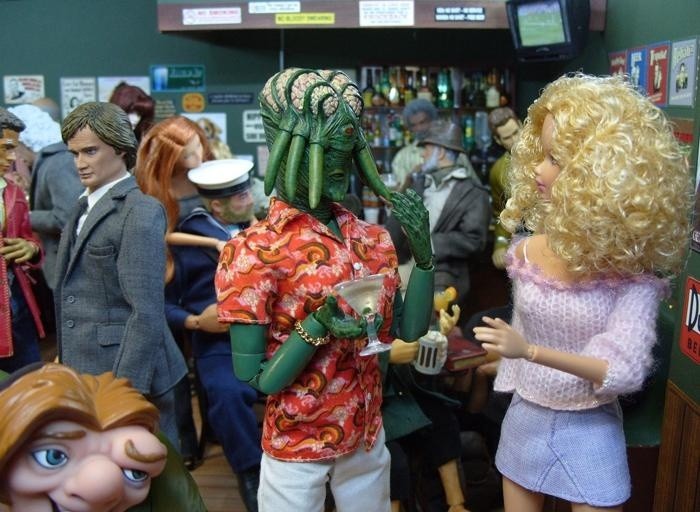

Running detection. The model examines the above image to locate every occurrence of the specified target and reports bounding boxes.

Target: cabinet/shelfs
[359,104,496,158]
